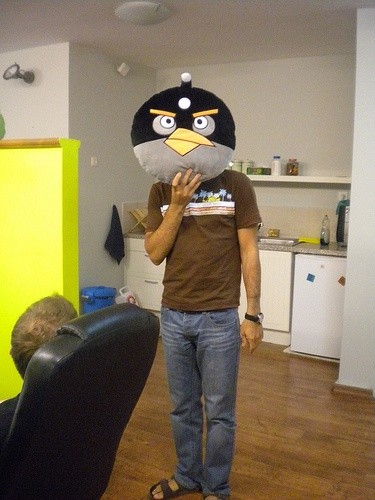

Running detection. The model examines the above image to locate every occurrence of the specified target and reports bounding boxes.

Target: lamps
[114,0,169,24]
[3,64,34,83]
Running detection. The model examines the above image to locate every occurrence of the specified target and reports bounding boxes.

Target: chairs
[0,303,161,500]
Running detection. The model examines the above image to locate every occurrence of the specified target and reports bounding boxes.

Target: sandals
[149,475,193,500]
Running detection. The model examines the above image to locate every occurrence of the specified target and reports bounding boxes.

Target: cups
[287,162,298,176]
[231,160,242,172]
[247,168,271,175]
[242,161,252,175]
[269,229,279,237]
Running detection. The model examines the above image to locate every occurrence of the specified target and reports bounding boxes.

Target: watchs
[245,312,264,325]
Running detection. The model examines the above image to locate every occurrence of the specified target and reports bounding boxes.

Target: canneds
[286,158,298,175]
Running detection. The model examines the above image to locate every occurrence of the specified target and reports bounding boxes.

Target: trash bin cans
[81,286,117,313]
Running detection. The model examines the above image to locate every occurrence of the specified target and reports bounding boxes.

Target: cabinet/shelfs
[123,237,165,310]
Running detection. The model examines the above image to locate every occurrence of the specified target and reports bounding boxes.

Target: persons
[144,169,264,500]
[0,293,78,458]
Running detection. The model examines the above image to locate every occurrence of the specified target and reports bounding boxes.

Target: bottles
[271,155,282,176]
[320,215,330,245]
